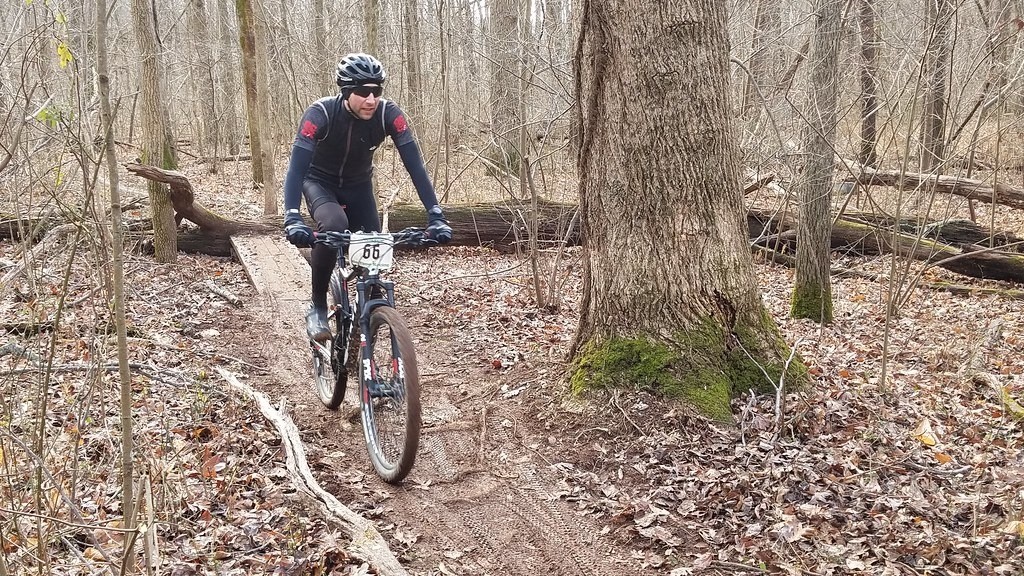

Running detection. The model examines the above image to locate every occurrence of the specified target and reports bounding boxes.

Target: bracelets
[285,208,300,216]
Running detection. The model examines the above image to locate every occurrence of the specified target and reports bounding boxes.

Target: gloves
[285,220,314,248]
[425,220,453,243]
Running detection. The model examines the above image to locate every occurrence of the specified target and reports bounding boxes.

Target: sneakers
[305,308,333,340]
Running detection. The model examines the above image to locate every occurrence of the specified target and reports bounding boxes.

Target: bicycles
[305,227,445,482]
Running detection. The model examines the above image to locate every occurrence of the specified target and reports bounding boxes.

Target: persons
[282,51,452,340]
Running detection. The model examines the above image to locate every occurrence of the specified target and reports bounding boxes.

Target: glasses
[340,85,383,97]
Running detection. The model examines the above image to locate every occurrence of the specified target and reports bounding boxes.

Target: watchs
[429,205,445,215]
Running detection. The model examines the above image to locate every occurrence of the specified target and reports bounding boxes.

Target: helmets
[336,52,386,85]
